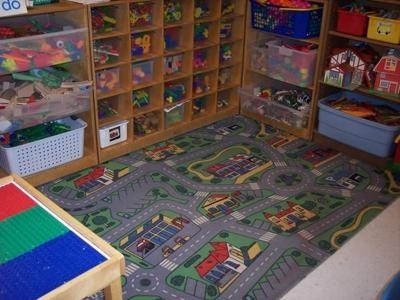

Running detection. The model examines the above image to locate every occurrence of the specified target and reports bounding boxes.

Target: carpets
[34,112,400,300]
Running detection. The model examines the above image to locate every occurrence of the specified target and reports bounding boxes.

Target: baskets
[250,0,322,40]
[0,114,88,178]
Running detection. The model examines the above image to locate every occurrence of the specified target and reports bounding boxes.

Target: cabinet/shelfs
[0,0,97,192]
[239,0,400,166]
[86,0,246,164]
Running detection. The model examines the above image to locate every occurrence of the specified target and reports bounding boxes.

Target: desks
[1,171,125,300]
